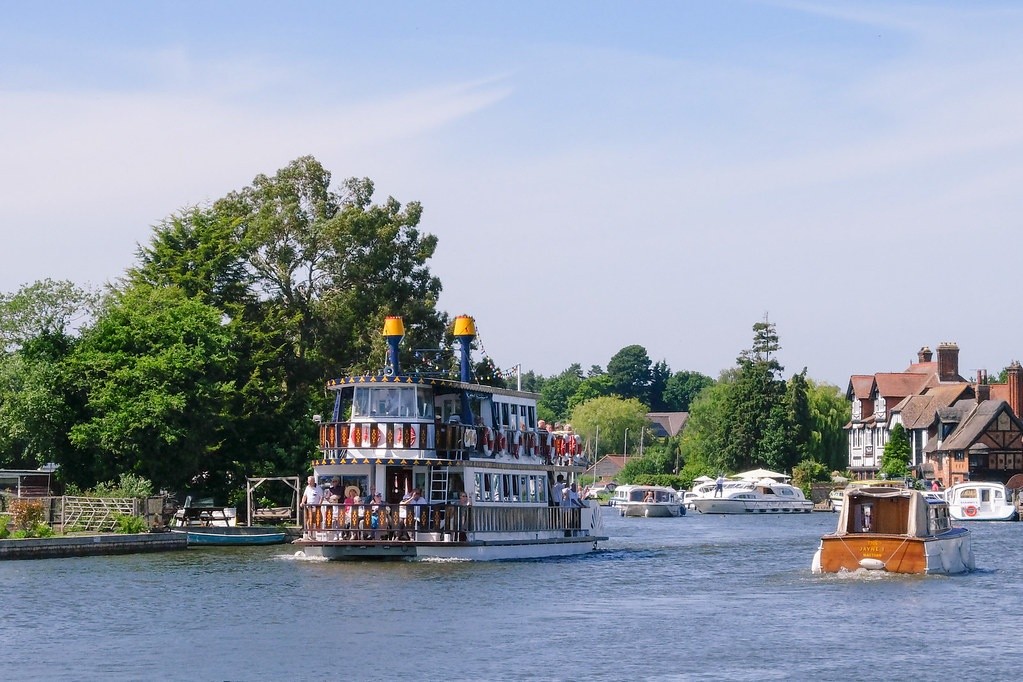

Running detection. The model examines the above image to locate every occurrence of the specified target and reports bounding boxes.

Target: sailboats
[583,424,610,499]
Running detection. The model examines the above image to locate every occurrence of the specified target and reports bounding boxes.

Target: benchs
[174,516,235,521]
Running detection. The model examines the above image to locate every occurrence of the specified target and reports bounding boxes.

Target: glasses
[461,496,467,497]
[378,496,381,497]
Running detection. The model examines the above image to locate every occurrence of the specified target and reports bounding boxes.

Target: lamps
[1004,465,1008,471]
[879,473,888,481]
[313,414,321,423]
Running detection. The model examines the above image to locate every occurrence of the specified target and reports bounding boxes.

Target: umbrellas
[729,468,790,482]
[693,476,713,492]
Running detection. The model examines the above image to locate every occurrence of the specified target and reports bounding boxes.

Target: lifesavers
[560,433,571,462]
[965,505,977,517]
[513,430,524,460]
[573,434,582,461]
[483,426,495,457]
[497,427,507,458]
[529,432,539,460]
[545,431,558,464]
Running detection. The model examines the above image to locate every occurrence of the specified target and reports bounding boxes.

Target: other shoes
[350,534,356,540]
[333,537,338,541]
[339,537,343,541]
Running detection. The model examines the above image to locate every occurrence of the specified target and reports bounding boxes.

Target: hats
[330,495,340,499]
[374,492,381,496]
[345,486,360,498]
[331,476,340,481]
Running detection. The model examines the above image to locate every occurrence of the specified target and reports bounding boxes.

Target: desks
[181,507,230,527]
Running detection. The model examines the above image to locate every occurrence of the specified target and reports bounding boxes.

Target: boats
[829,482,948,513]
[609,485,685,518]
[185,531,285,545]
[812,486,974,574]
[691,478,815,514]
[678,476,718,510]
[946,481,1015,521]
[293,313,610,560]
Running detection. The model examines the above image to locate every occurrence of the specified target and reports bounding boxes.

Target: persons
[552,473,586,527]
[535,420,573,464]
[932,481,939,491]
[714,475,723,498]
[644,492,655,503]
[298,473,470,540]
